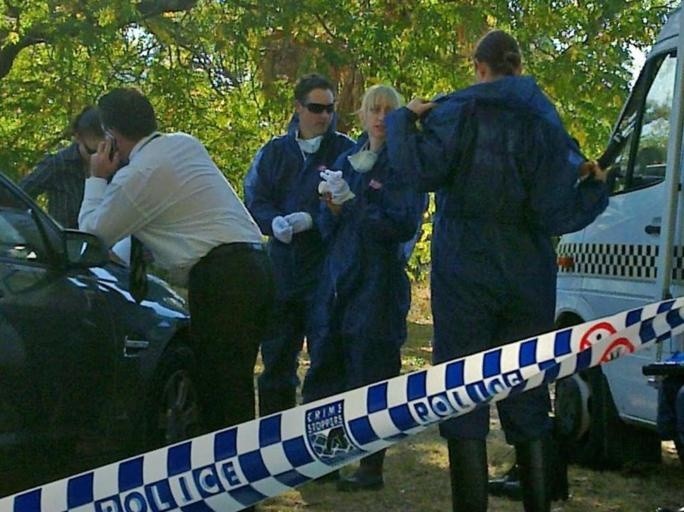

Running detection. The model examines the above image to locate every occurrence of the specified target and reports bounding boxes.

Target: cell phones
[104,131,116,157]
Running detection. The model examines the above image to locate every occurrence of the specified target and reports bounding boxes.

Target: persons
[299,84,432,492]
[384,30,609,511]
[76,89,271,433]
[18,105,107,229]
[245,74,357,482]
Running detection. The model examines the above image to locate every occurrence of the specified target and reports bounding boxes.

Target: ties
[129,235,149,307]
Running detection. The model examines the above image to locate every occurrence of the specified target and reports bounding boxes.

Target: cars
[1,170,195,460]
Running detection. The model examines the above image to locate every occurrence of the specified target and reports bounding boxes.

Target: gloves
[318,170,356,204]
[272,212,313,245]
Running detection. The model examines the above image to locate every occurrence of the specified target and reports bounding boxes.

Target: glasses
[81,141,97,155]
[301,103,334,114]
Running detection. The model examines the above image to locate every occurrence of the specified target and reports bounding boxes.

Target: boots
[315,448,385,492]
[448,438,487,511]
[518,440,550,511]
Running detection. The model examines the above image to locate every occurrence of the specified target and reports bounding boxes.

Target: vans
[551,0,683,468]
